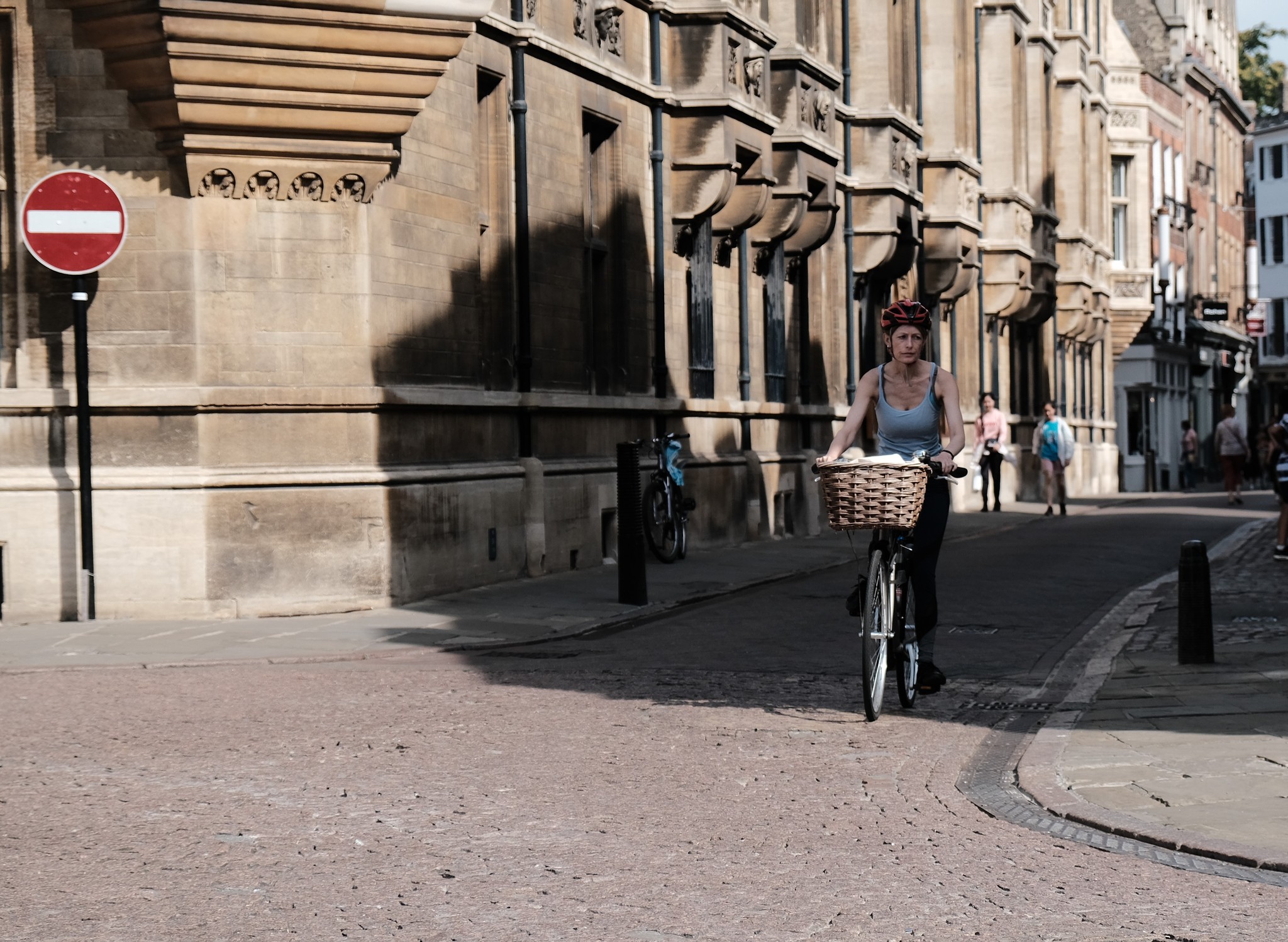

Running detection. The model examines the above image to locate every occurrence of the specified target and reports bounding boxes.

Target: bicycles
[632,434,691,563]
[812,459,969,720]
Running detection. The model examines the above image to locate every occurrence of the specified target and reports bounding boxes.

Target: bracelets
[942,450,954,459]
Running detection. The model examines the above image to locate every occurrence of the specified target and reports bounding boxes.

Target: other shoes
[916,658,946,693]
[845,575,867,617]
[1060,505,1066,516]
[992,502,1001,512]
[1045,506,1053,516]
[1273,546,1288,559]
[980,505,988,512]
[1235,498,1243,504]
[1228,499,1234,504]
[1181,484,1195,489]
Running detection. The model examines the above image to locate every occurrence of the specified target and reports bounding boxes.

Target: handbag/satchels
[1031,455,1040,471]
[985,439,998,450]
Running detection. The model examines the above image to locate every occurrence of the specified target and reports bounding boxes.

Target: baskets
[817,460,933,531]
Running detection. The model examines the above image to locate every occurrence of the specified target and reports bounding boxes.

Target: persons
[817,299,965,686]
[1179,404,1288,560]
[1031,401,1074,517]
[973,392,1007,512]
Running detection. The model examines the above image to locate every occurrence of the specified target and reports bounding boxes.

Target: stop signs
[19,169,129,275]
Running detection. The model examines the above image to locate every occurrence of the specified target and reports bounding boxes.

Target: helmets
[881,299,932,333]
